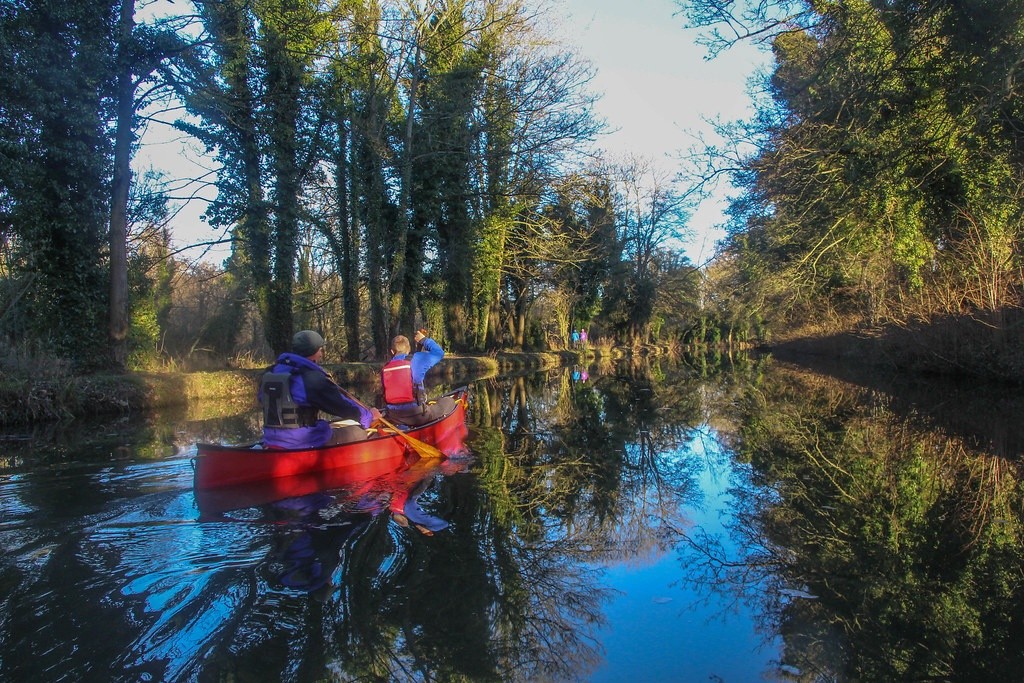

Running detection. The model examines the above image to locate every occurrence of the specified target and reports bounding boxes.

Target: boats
[192,385,470,514]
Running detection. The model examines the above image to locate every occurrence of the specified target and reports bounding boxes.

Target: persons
[257,330,382,450]
[381,331,454,426]
[580,328,587,350]
[572,329,580,349]
[580,372,589,384]
[572,371,581,383]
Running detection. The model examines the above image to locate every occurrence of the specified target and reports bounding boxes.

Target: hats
[291,330,328,356]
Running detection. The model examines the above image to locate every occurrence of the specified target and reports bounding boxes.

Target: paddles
[335,382,449,459]
[416,329,428,352]
